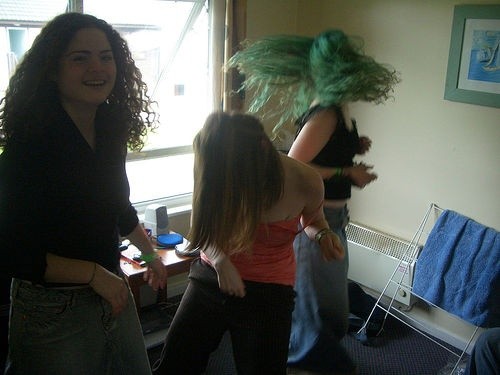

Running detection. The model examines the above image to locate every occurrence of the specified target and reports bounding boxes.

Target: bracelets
[87,263,96,284]
[315,228,333,245]
[335,168,343,177]
[139,252,157,265]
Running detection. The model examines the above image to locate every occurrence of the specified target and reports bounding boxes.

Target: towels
[411,207,500,330]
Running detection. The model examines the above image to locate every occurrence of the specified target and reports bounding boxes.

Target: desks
[116,215,197,318]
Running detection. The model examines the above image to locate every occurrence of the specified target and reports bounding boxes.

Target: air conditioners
[343,221,424,306]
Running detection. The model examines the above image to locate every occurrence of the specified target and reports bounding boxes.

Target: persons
[220,27,402,367]
[0,12,168,374]
[153,110,345,375]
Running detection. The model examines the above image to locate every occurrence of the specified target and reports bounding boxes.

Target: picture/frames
[443,4,500,110]
